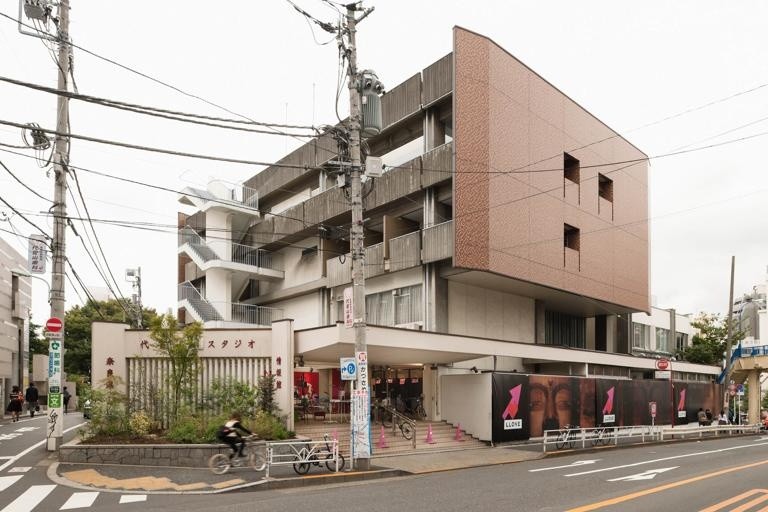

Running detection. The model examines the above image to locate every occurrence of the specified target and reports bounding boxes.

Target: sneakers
[229,454,248,460]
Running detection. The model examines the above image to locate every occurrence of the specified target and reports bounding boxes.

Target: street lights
[736,298,767,357]
[9,3,70,452]
[126,264,144,328]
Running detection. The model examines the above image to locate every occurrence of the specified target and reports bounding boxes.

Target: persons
[217,409,258,460]
[697,407,714,426]
[528,383,574,436]
[718,410,728,434]
[26,382,39,417]
[6,385,24,422]
[63,386,72,413]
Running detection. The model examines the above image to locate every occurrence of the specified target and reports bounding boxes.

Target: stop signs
[46,318,62,332]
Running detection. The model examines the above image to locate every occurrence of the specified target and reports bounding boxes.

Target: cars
[759,406,768,430]
[83,399,92,418]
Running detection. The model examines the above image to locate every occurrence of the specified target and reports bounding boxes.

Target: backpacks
[216,426,230,437]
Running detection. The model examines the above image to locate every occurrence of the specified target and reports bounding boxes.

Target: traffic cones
[426,424,436,444]
[375,425,390,448]
[453,422,464,441]
[328,428,341,452]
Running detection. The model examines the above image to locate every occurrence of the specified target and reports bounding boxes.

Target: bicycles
[209,434,265,473]
[590,422,612,446]
[399,392,426,420]
[556,424,577,449]
[293,433,345,474]
[383,407,415,441]
